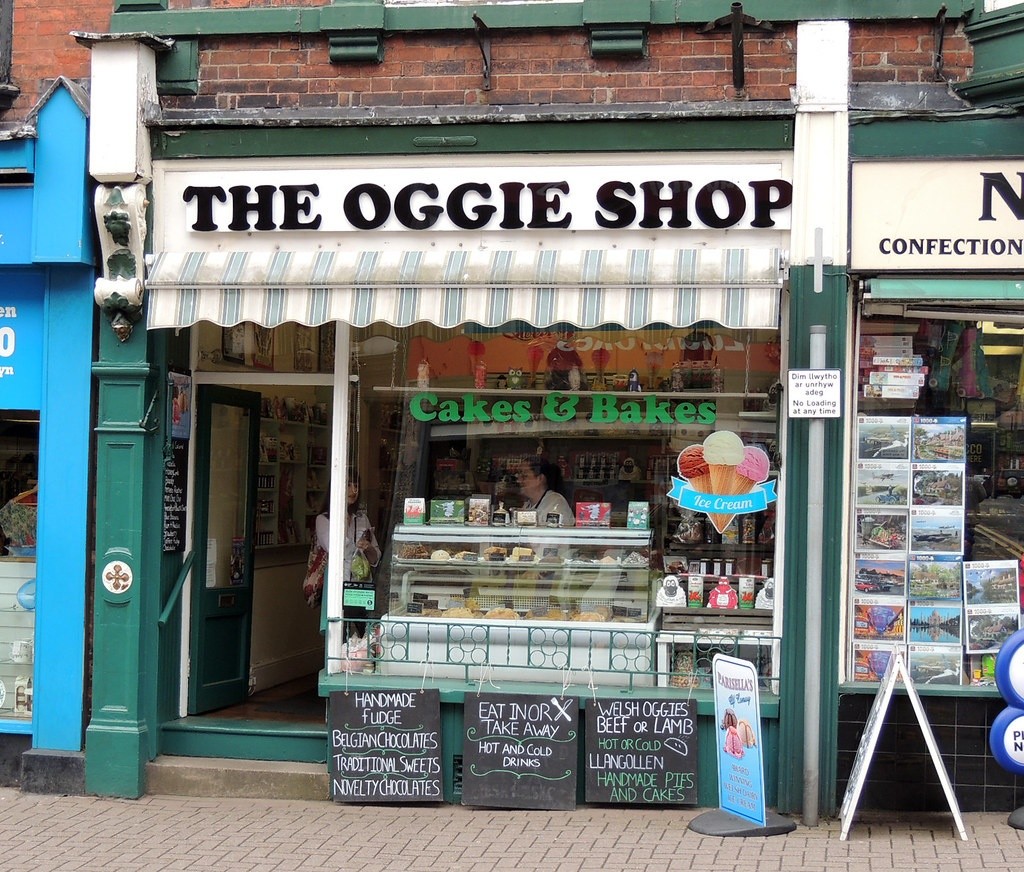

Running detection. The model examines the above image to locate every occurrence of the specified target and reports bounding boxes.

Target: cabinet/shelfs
[236,372,1024,695]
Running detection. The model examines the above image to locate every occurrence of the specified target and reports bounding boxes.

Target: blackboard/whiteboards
[584,697,700,806]
[329,689,444,802]
[461,692,579,810]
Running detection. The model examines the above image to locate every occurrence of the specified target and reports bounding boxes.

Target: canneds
[700,558,773,578]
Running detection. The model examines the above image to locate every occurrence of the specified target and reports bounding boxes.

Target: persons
[516,454,574,560]
[315,466,381,642]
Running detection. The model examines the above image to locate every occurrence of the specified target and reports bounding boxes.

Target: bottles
[475,360,487,388]
[417,359,429,388]
[629,368,638,392]
[558,453,619,480]
[671,361,724,393]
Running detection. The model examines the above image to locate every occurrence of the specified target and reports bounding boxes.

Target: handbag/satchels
[302,511,329,609]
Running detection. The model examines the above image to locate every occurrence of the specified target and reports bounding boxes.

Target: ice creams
[677,431,769,534]
[721,708,756,757]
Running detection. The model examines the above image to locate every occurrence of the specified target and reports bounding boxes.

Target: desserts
[399,545,649,566]
